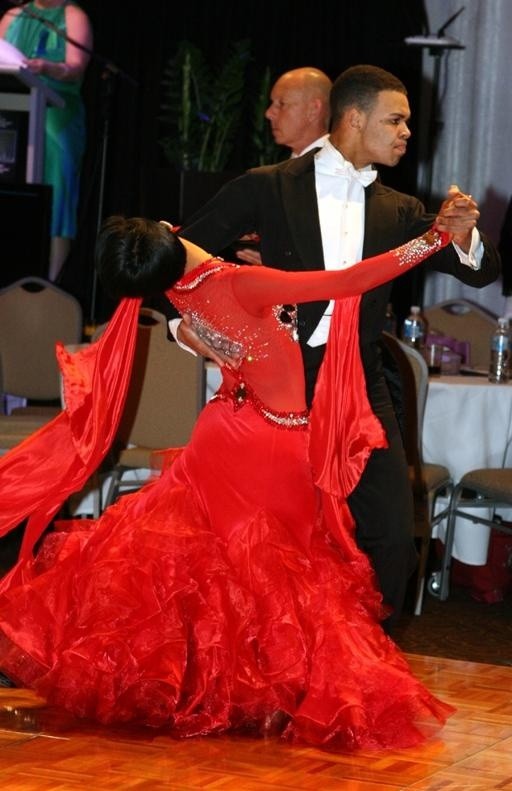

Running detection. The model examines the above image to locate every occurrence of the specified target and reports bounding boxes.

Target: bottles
[402,305,426,352]
[420,329,444,374]
[489,317,510,385]
[383,303,397,337]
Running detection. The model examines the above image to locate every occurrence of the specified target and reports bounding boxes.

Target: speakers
[0,183,52,292]
[180,171,247,225]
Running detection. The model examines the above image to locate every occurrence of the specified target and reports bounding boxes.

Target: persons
[0,0,95,284]
[0,184,479,756]
[163,65,500,630]
[235,67,333,266]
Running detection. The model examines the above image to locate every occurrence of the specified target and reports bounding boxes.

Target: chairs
[383,297,512,618]
[1,277,205,521]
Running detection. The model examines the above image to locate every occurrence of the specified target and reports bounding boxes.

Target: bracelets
[55,62,70,81]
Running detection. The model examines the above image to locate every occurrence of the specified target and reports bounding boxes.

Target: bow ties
[315,159,378,189]
